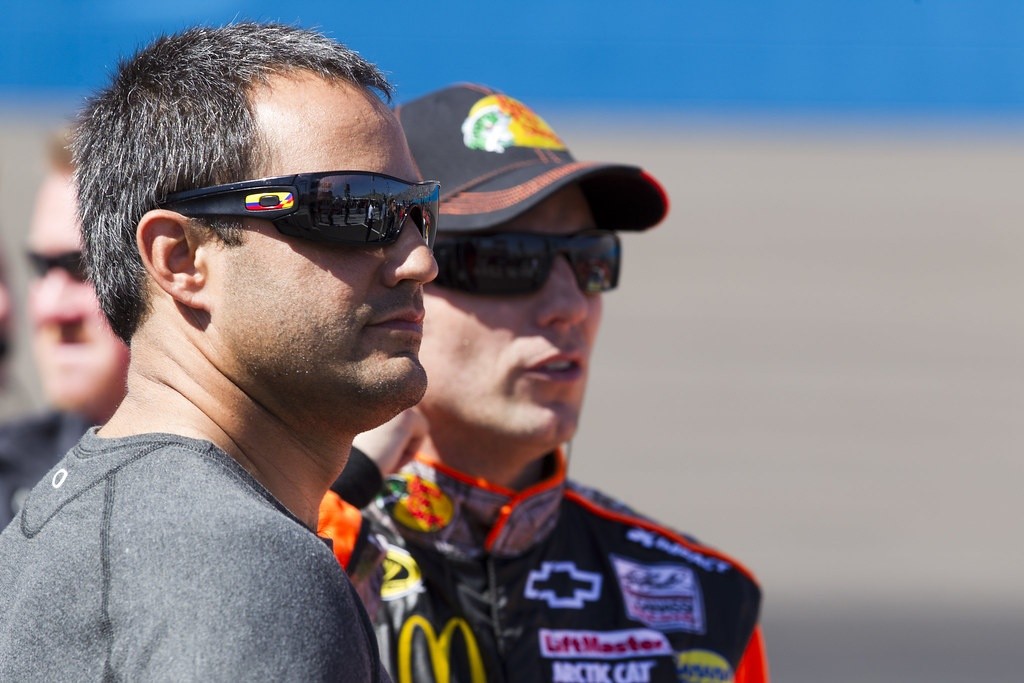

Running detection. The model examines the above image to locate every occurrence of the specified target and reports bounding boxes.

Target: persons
[0,21,439,683]
[309,200,324,227]
[344,203,350,225]
[327,200,334,226]
[364,199,410,241]
[314,84,769,683]
[575,254,610,291]
[1,121,128,530]
[531,255,539,285]
[421,202,430,239]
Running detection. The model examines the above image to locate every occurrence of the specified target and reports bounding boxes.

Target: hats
[392,85,668,235]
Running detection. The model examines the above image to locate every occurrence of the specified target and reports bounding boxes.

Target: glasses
[147,172,440,252]
[431,228,620,299]
[22,242,90,282]
